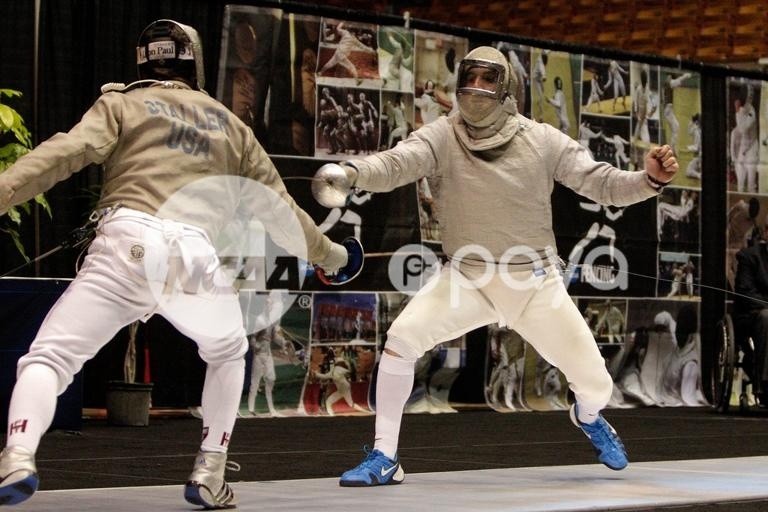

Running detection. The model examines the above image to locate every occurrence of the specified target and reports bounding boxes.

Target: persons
[735,210,768,404]
[0,17,678,509]
[653,72,759,405]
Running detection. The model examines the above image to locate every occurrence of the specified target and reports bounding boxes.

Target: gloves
[338,164,357,189]
[317,239,350,277]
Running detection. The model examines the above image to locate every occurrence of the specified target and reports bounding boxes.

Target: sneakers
[0,446,38,504]
[568,403,629,471]
[339,449,405,486]
[184,448,240,508]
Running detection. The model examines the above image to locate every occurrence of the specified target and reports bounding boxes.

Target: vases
[103,378,157,428]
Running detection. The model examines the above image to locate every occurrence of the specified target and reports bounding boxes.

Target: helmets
[135,19,206,91]
[692,113,701,122]
[541,51,549,65]
[554,76,563,90]
[455,45,517,124]
[641,71,648,88]
